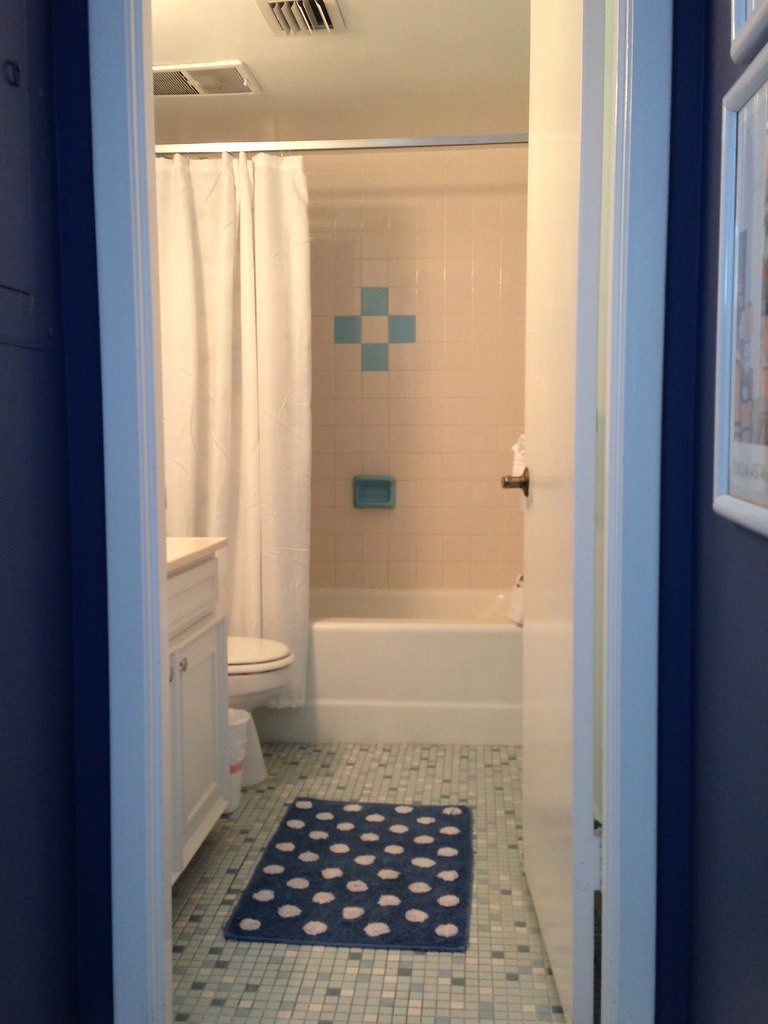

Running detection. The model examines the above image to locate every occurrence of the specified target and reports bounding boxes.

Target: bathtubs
[251,587,524,747]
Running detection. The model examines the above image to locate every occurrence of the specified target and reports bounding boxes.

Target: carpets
[221,798,474,953]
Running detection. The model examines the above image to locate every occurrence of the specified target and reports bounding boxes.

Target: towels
[507,574,525,625]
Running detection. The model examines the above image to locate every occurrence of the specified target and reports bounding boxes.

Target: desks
[166,535,230,889]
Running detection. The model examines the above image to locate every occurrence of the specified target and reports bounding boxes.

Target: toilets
[226,636,296,789]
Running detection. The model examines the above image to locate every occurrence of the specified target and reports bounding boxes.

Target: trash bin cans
[222,708,251,815]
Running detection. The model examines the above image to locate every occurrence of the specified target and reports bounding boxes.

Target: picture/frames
[711,0,768,535]
